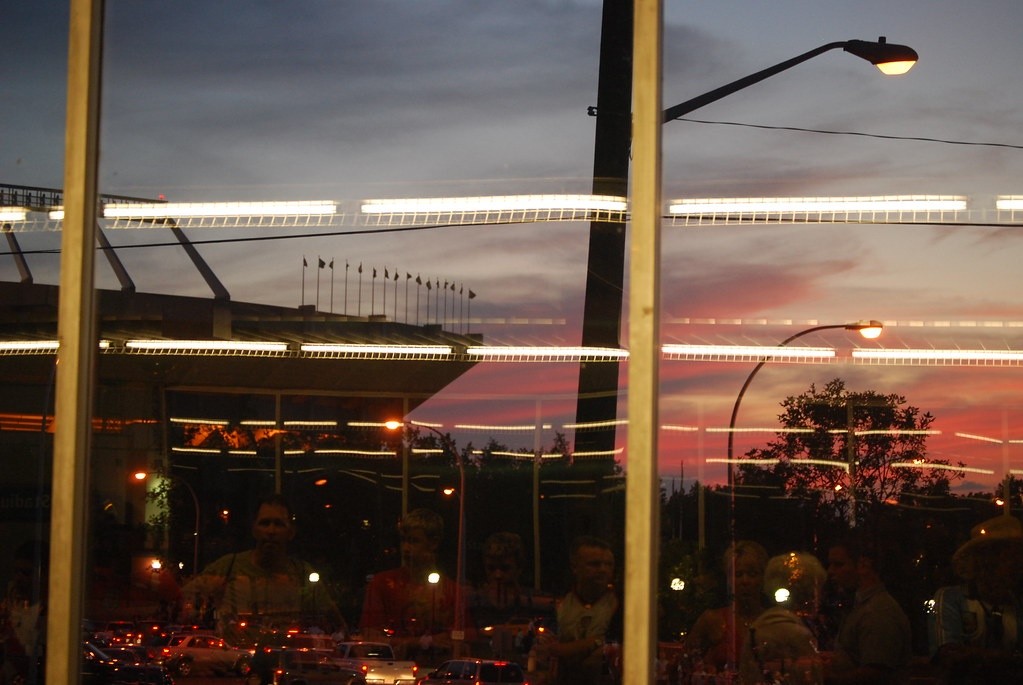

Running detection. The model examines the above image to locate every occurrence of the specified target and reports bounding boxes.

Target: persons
[0,494,1023,685]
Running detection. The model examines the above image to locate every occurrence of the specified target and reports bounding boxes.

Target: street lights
[308,572,320,625]
[571,31,921,472]
[425,570,442,663]
[384,416,465,659]
[134,466,201,608]
[724,318,885,668]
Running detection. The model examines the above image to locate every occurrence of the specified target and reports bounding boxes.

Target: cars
[82,602,688,684]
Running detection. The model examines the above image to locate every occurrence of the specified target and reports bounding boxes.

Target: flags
[304,258,399,280]
[406,274,476,298]
